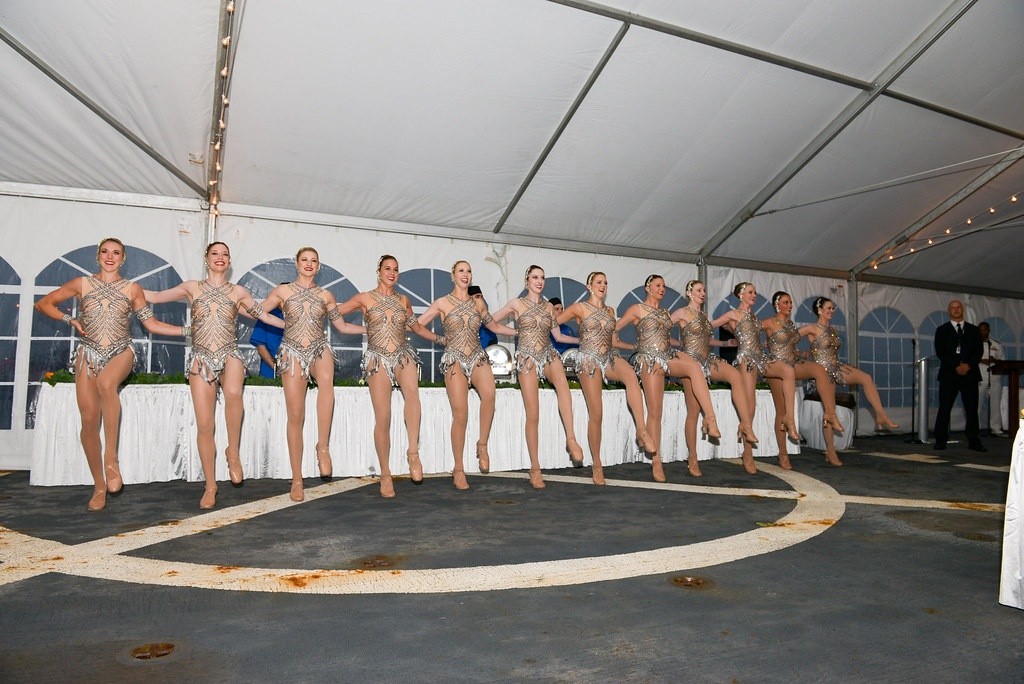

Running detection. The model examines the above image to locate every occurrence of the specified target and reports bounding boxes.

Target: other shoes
[933,430,1009,452]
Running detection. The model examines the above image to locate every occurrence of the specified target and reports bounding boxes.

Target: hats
[468,284,481,295]
[549,297,562,306]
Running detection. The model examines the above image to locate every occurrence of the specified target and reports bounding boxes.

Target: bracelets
[61,314,74,326]
[181,325,192,338]
[434,334,444,345]
[722,341,728,347]
[633,343,638,351]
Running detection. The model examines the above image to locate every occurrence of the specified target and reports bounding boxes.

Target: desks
[27,382,803,483]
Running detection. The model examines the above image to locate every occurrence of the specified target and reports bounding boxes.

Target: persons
[249,282,288,379]
[934,301,988,453]
[615,275,720,483]
[33,237,192,510]
[405,259,517,491]
[974,320,1005,438]
[711,283,803,475]
[797,297,898,466]
[491,265,581,489]
[762,288,844,470]
[673,280,759,477]
[557,270,656,486]
[466,285,499,350]
[141,242,285,510]
[549,298,580,354]
[238,248,369,501]
[338,255,446,497]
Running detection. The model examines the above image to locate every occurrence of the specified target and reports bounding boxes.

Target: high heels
[379,475,395,498]
[452,470,469,490]
[88,486,106,511]
[316,443,333,478]
[289,479,304,501]
[702,417,721,438]
[103,462,122,493]
[739,414,896,475]
[592,467,606,486]
[566,439,583,463]
[200,483,218,509]
[477,441,489,472]
[653,462,666,482]
[225,447,242,484]
[637,430,656,453]
[407,451,423,482]
[529,469,544,489]
[687,457,702,476]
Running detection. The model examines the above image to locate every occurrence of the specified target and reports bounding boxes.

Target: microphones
[986,339,991,345]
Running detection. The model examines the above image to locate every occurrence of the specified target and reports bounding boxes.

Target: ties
[957,323,963,347]
